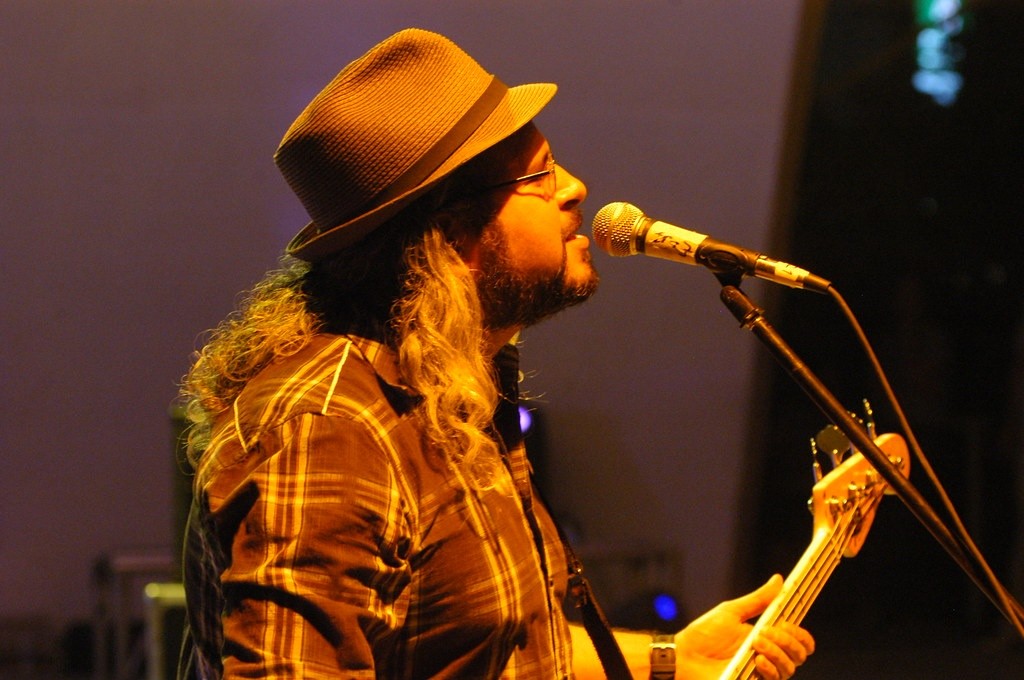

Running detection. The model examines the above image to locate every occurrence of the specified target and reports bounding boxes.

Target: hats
[272,26,559,265]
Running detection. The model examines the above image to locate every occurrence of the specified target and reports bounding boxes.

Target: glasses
[433,160,557,217]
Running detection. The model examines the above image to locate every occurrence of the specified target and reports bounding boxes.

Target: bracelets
[648,632,676,680]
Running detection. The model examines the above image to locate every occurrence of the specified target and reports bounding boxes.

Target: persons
[174,28,815,680]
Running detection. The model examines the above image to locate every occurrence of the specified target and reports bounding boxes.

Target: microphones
[591,200,834,294]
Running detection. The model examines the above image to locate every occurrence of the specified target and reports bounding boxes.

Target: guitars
[718,397,913,680]
